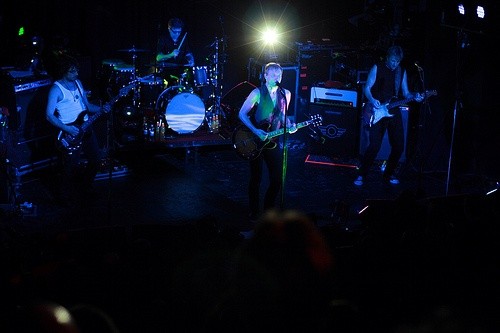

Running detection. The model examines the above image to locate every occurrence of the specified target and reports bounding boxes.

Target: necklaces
[62,79,76,102]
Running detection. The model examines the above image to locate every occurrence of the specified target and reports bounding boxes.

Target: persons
[154,17,195,67]
[355,44,423,187]
[238,63,292,209]
[0,210,500,333]
[46,53,112,208]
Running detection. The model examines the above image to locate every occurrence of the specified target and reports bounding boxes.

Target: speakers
[2,86,56,164]
[305,103,359,161]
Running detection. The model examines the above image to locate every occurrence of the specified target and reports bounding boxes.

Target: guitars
[232,114,324,161]
[56,80,138,157]
[363,88,440,127]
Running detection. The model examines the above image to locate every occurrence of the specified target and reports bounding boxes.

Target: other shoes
[250,211,256,223]
[352,175,366,188]
[384,175,400,186]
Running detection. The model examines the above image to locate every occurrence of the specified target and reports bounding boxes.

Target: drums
[184,66,209,89]
[155,85,206,135]
[136,79,162,103]
[103,60,125,75]
[115,67,136,86]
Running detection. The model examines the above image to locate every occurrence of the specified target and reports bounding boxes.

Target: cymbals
[143,63,183,68]
[115,48,153,52]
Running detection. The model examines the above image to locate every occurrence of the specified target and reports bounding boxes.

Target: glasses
[67,68,80,74]
[170,28,182,34]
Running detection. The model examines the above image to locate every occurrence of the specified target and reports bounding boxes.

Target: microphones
[276,81,285,93]
[414,61,425,72]
